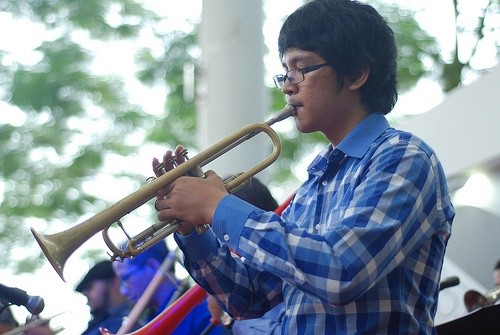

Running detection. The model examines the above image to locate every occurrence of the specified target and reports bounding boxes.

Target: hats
[74,259,115,292]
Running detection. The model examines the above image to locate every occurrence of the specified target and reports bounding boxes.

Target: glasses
[274,62,327,90]
[120,265,139,280]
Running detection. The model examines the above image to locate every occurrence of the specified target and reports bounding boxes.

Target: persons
[491,260,500,285]
[0,172,283,335]
[152,0,455,335]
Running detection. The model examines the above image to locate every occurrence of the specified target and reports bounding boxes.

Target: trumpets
[25,103,297,282]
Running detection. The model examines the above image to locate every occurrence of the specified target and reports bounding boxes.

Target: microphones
[440,276,460,291]
[0,283,45,316]
